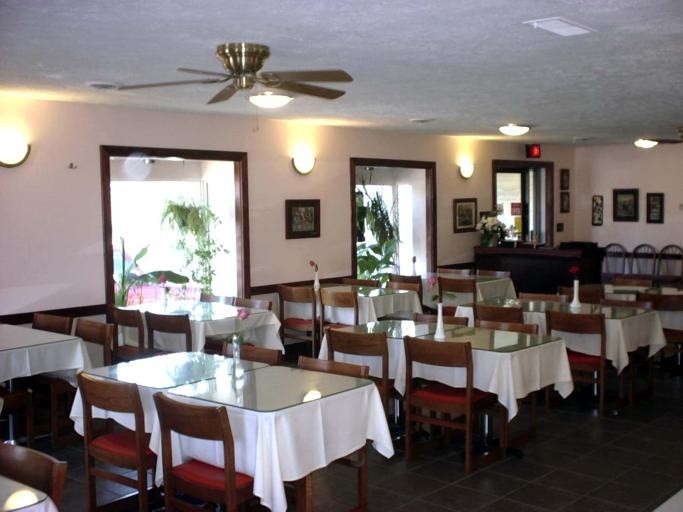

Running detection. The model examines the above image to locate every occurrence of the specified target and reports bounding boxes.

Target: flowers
[475,214,501,246]
[309,260,317,268]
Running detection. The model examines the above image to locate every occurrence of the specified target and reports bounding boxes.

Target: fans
[118,41,354,105]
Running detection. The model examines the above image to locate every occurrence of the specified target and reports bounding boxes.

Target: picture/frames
[646,192,664,223]
[612,188,639,222]
[453,197,477,233]
[561,169,569,190]
[592,194,603,226]
[560,192,569,213]
[285,198,320,238]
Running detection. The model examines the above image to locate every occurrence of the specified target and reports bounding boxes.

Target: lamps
[499,123,531,136]
[634,138,659,149]
[292,144,316,175]
[0,127,31,167]
[459,159,475,179]
[246,89,294,109]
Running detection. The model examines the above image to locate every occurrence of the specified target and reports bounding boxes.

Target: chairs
[629,244,656,275]
[600,242,626,282]
[657,245,683,276]
